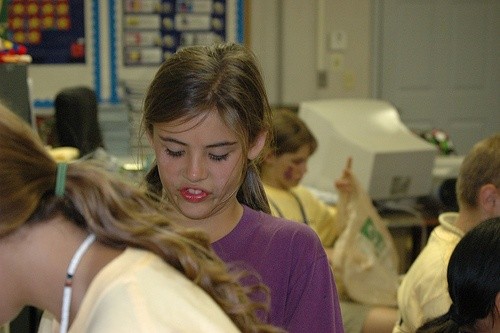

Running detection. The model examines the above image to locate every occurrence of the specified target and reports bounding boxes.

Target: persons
[0,43,500,333]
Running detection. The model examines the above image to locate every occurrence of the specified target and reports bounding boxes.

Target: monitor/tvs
[295,100,436,199]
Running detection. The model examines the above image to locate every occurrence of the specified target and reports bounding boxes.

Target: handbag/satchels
[333,171,399,308]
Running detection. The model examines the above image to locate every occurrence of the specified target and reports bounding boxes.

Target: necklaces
[264,189,308,225]
[59,234,95,333]
